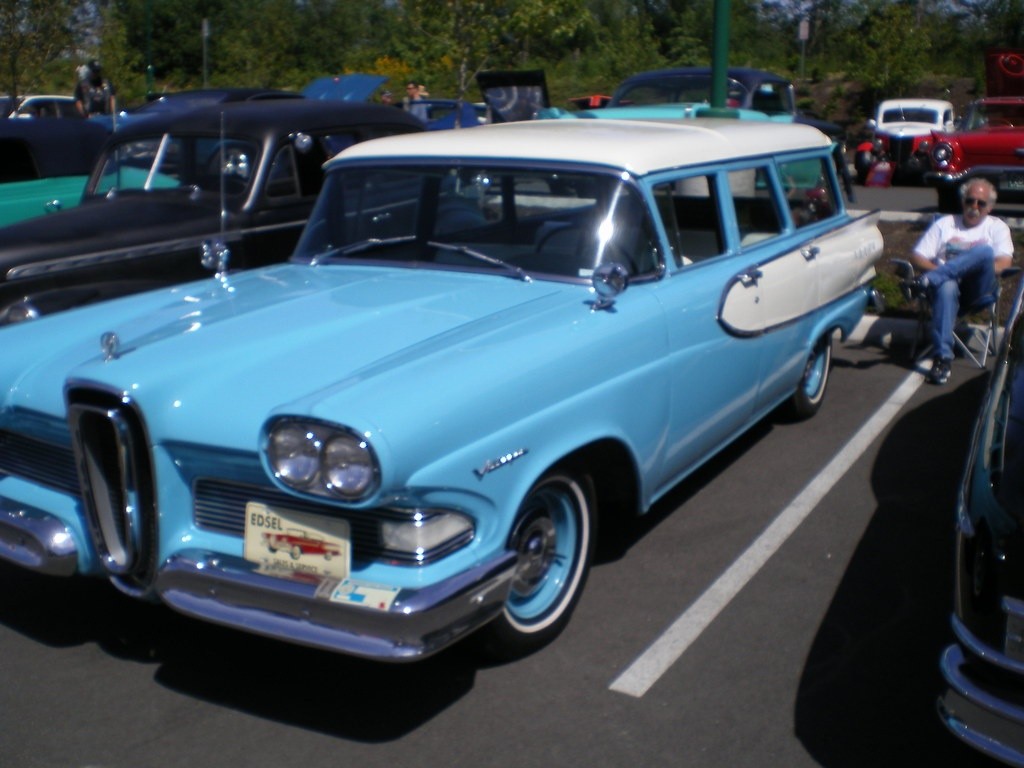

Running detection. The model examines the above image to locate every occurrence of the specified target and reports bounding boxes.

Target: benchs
[535,220,776,264]
[435,243,644,279]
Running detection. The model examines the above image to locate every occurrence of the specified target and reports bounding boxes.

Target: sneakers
[898,277,923,304]
[926,357,951,384]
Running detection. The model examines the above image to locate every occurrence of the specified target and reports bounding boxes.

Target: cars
[932,274,1024,768]
[913,95,1024,209]
[851,93,960,189]
[0,59,852,331]
[0,109,888,668]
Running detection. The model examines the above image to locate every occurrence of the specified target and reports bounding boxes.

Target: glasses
[965,198,986,209]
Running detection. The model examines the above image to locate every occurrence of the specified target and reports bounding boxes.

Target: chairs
[887,212,1024,371]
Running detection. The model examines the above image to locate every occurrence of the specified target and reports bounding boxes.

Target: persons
[380,82,430,122]
[76,61,117,120]
[896,180,1014,385]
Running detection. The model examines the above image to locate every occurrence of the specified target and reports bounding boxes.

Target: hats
[88,60,101,71]
[380,90,391,97]
[418,85,429,96]
